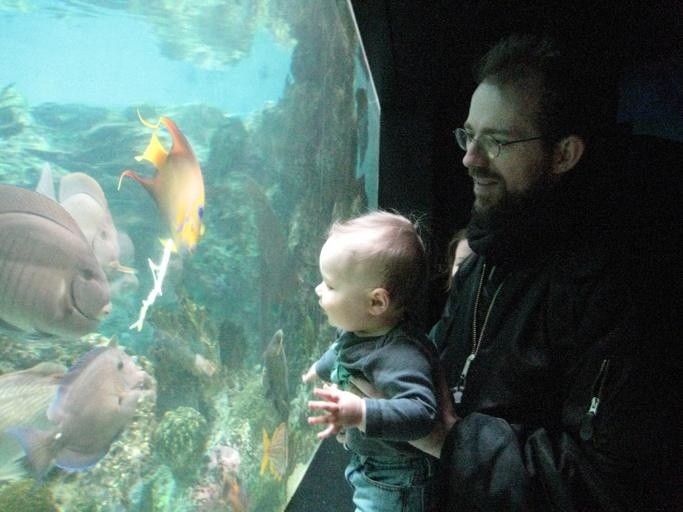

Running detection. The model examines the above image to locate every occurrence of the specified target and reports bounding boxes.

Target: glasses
[455,127,543,159]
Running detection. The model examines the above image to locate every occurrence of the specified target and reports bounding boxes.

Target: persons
[447,224,474,280]
[301,209,443,511]
[346,30,681,512]
